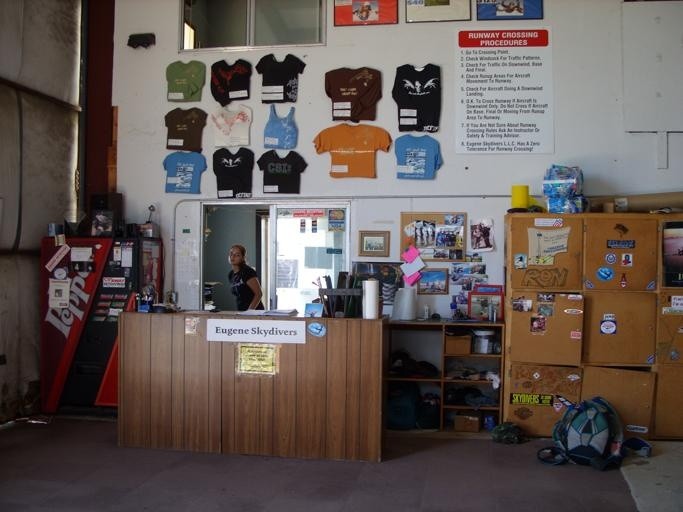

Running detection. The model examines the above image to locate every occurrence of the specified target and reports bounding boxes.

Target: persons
[435,230,462,246]
[227,244,265,311]
[473,224,487,247]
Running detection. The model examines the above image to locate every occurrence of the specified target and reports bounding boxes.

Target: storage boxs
[445,330,471,353]
[470,326,494,355]
[455,410,483,431]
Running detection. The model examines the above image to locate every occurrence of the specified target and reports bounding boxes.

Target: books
[239,306,299,316]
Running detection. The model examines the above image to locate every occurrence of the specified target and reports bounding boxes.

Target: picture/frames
[468,292,503,319]
[474,284,503,293]
[417,268,448,295]
[357,229,389,258]
[332,0,544,28]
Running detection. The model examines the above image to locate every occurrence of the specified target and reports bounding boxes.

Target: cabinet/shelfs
[501,209,681,443]
[384,318,504,440]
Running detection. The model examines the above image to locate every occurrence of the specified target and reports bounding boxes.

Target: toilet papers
[393,288,418,321]
[361,280,380,320]
